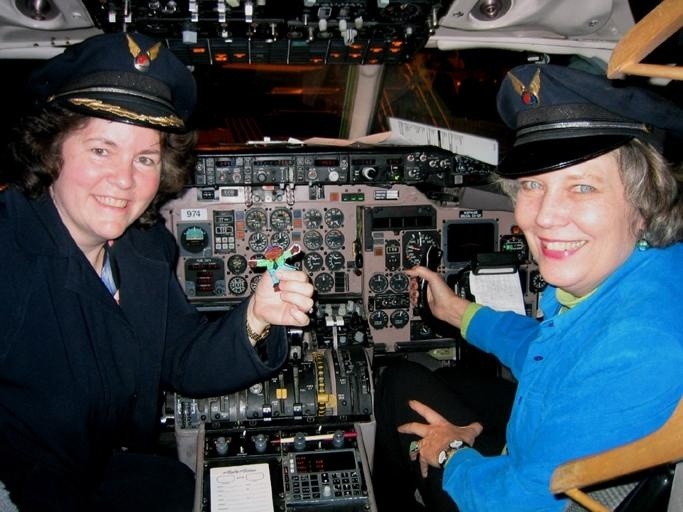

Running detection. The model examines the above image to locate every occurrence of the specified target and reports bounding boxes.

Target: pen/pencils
[270,431,357,443]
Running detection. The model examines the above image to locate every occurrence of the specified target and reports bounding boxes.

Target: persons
[0,31,314,512]
[394,60,683,512]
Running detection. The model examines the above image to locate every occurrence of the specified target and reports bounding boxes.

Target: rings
[409,440,419,453]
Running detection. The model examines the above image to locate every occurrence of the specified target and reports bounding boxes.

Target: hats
[26,31,199,135]
[493,62,682,179]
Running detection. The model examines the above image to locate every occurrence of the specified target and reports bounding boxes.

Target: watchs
[437,448,451,469]
[445,438,471,451]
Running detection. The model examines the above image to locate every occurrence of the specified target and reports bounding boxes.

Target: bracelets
[245,321,271,341]
